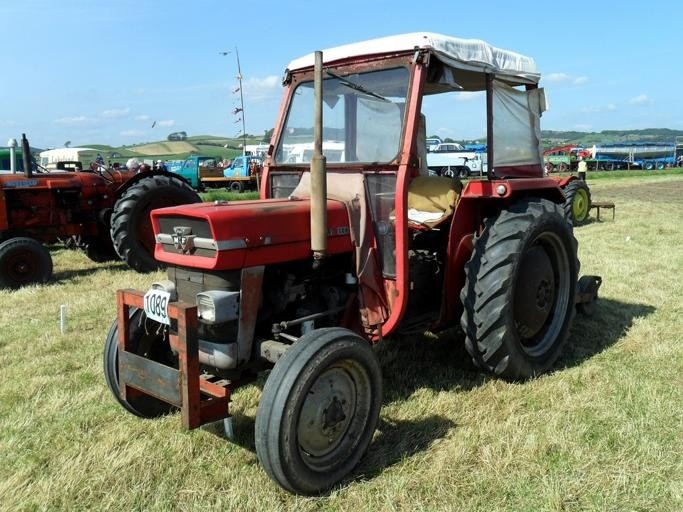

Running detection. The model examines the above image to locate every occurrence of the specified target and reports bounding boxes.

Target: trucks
[571,143,678,171]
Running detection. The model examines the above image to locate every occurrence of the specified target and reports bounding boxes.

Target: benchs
[589,202,615,220]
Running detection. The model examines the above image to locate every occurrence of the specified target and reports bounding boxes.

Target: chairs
[388,176,463,231]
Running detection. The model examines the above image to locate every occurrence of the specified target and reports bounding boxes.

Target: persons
[89,154,257,180]
[544,163,549,176]
[577,156,587,180]
